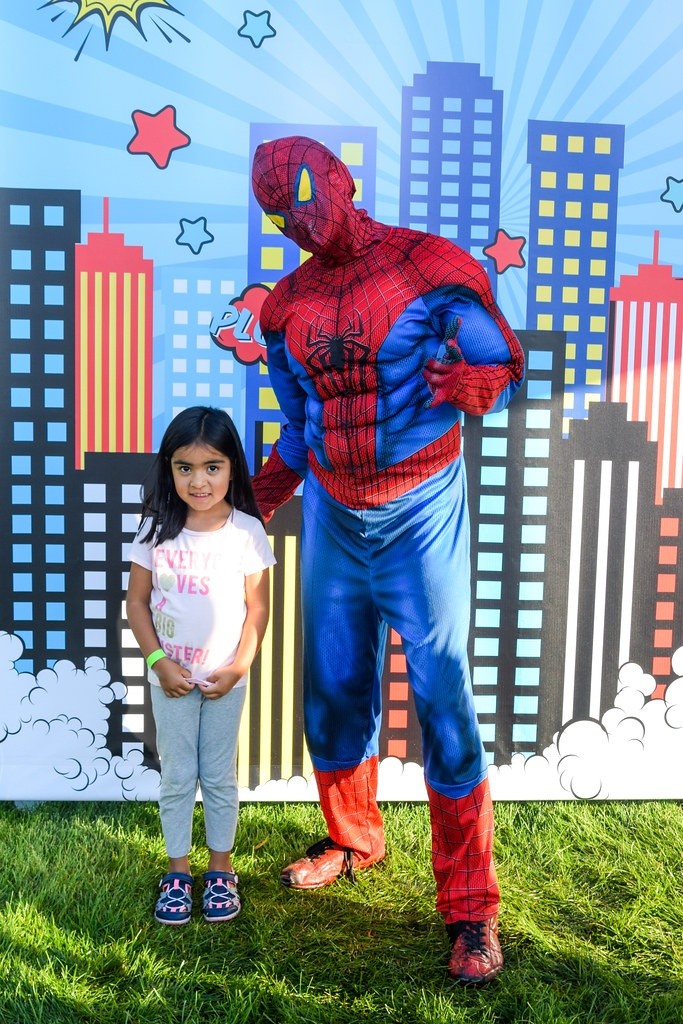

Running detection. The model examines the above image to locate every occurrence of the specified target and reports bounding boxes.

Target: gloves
[420,316,467,410]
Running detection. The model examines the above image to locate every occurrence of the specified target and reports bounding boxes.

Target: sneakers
[280,836,387,889]
[447,911,504,984]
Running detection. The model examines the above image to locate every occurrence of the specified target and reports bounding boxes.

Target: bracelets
[146,649,166,669]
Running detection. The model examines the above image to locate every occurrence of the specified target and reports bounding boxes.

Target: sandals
[154,872,195,925]
[201,866,242,921]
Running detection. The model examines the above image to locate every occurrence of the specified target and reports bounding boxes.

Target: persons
[124,406,277,925]
[252,137,525,987]
